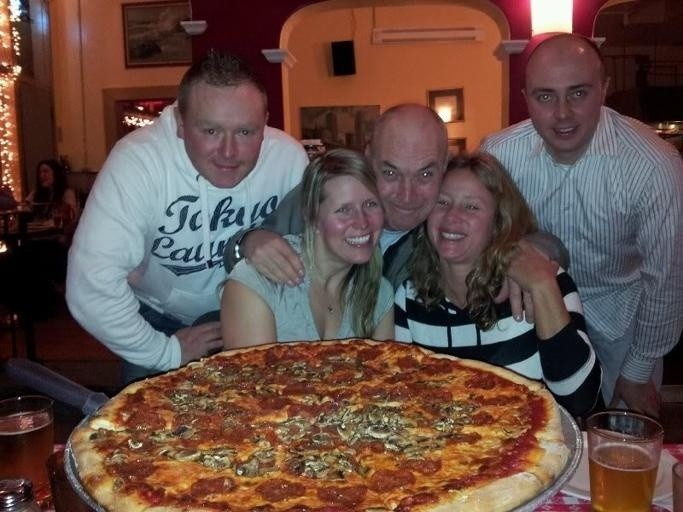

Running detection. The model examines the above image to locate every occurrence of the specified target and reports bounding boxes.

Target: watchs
[235,233,247,263]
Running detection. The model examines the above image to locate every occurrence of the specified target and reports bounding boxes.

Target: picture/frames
[427,89,465,125]
[102,85,178,158]
[447,138,467,159]
[122,1,193,69]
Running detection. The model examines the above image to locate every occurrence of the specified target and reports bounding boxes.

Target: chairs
[0,212,40,364]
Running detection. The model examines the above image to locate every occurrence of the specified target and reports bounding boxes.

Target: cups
[586,411,663,512]
[0,397,56,508]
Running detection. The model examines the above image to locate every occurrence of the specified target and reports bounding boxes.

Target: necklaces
[312,278,347,315]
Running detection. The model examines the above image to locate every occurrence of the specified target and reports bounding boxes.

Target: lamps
[372,26,481,42]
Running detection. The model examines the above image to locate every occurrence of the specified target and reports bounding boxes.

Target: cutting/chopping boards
[6,346,581,512]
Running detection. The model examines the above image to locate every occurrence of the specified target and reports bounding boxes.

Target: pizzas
[69,337,571,512]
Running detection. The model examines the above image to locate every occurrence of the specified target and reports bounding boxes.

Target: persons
[393,150,604,421]
[22,157,81,218]
[472,33,683,434]
[225,101,570,323]
[220,148,394,353]
[64,47,311,388]
